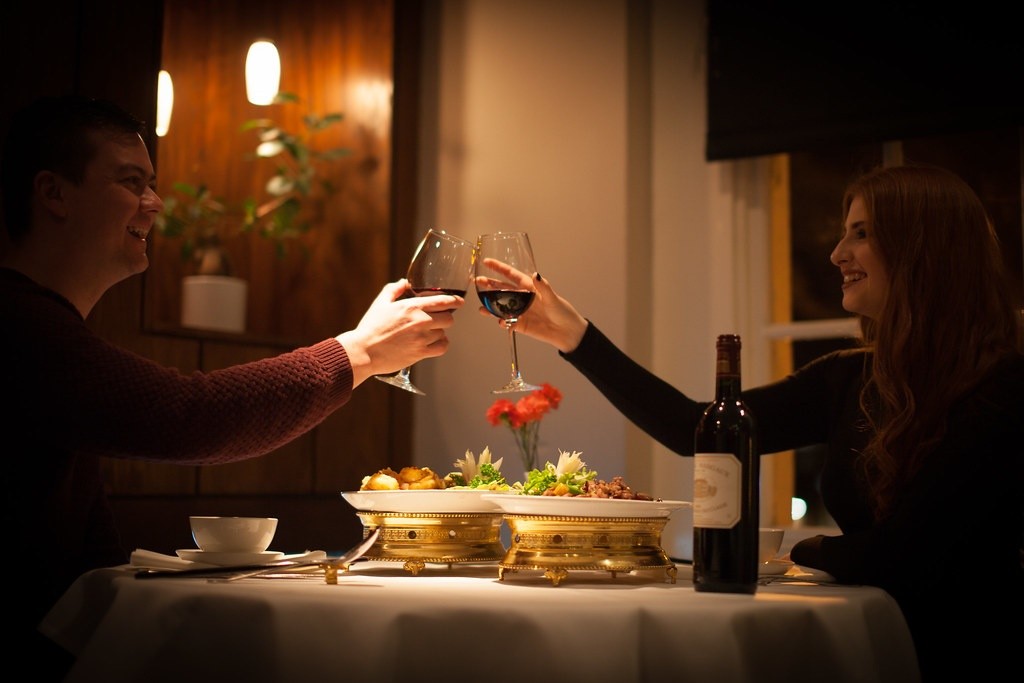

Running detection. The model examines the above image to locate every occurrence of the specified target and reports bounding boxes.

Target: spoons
[136,527,382,579]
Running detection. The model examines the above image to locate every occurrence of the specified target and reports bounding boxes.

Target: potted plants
[153,93,379,330]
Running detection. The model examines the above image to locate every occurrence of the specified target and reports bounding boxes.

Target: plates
[176,549,285,566]
[758,561,795,575]
[481,493,692,518]
[342,491,519,512]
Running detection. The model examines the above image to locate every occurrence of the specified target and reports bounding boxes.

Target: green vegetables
[468,464,506,490]
[517,460,598,496]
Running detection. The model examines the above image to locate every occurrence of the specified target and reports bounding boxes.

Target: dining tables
[62,561,917,683]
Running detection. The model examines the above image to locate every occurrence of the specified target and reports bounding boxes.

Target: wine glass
[375,229,478,396]
[475,232,544,394]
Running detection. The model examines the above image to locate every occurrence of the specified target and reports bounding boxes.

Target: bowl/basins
[190,516,278,552]
[759,528,784,562]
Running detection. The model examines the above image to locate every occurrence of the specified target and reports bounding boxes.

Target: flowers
[487,383,562,474]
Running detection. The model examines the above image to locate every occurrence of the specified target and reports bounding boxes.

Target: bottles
[693,334,761,593]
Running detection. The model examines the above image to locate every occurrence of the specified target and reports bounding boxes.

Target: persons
[479,160,1024,683]
[0,90,467,683]
